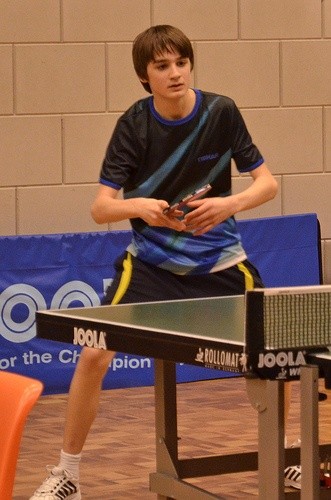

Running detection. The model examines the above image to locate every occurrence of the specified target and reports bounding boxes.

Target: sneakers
[285,439,331,490]
[29,465,82,500]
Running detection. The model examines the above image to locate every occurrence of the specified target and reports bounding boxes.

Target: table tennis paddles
[163,183,215,217]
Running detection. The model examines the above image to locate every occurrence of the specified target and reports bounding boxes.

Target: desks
[35,285,331,500]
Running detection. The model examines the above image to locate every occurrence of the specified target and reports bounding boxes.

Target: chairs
[0,370,44,500]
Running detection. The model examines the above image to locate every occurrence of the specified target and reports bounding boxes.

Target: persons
[30,24,331,500]
